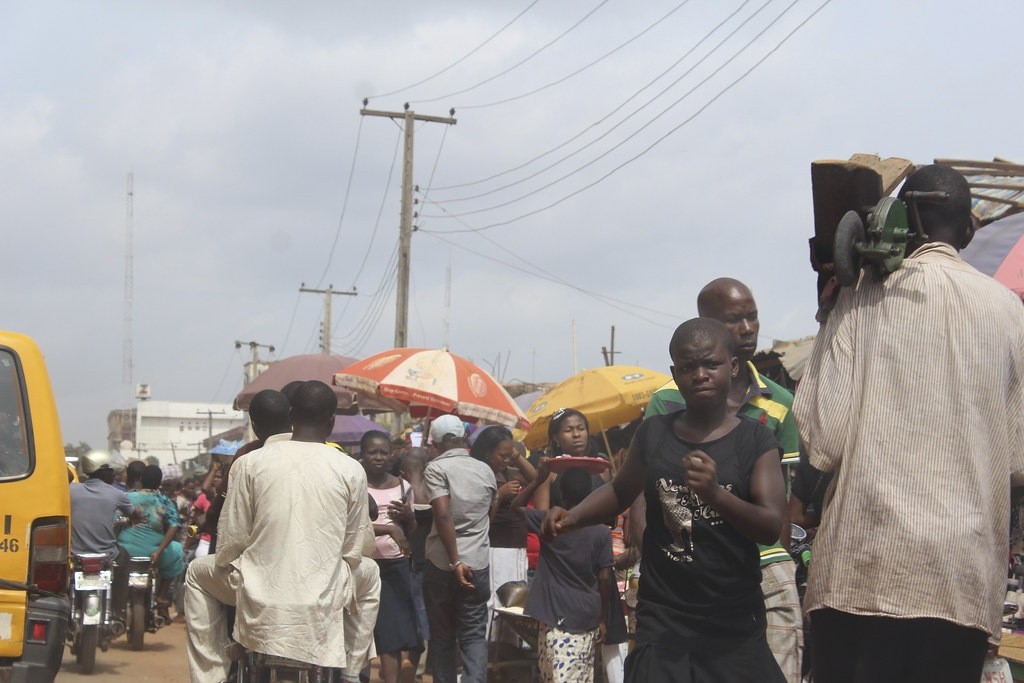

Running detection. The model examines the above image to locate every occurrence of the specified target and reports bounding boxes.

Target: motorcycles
[231,605,335,683]
[68,549,126,674]
[126,555,164,652]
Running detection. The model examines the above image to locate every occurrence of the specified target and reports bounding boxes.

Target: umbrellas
[959,212,1024,300]
[332,346,532,448]
[511,366,672,476]
[326,415,389,443]
[232,354,395,416]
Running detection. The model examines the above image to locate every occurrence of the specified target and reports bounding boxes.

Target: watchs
[449,561,462,570]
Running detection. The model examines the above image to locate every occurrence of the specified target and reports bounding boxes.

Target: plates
[546,457,610,475]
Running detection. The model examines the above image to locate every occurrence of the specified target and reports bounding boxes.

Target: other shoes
[399,660,416,683]
[152,596,171,604]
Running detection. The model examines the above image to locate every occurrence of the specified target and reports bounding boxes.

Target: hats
[430,414,465,443]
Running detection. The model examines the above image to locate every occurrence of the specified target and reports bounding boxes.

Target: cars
[0,326,72,683]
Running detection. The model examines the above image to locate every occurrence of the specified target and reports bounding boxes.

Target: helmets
[80,449,117,474]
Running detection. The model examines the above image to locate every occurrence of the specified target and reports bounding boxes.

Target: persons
[114,465,183,625]
[423,414,497,683]
[792,165,1024,683]
[184,380,381,683]
[631,277,804,683]
[510,457,616,683]
[70,450,142,623]
[126,410,647,683]
[540,317,788,683]
